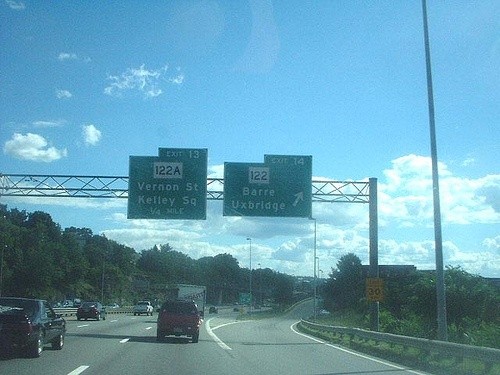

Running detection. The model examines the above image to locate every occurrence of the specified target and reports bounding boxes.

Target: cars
[157,299,200,343]
[48,297,120,311]
[77,302,106,321]
[232,299,272,312]
[209,307,218,314]
[0,297,66,358]
[134,301,153,316]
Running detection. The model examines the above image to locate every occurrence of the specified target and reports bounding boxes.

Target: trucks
[178,285,206,320]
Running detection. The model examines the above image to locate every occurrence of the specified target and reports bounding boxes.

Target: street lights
[309,218,317,323]
[246,237,251,294]
